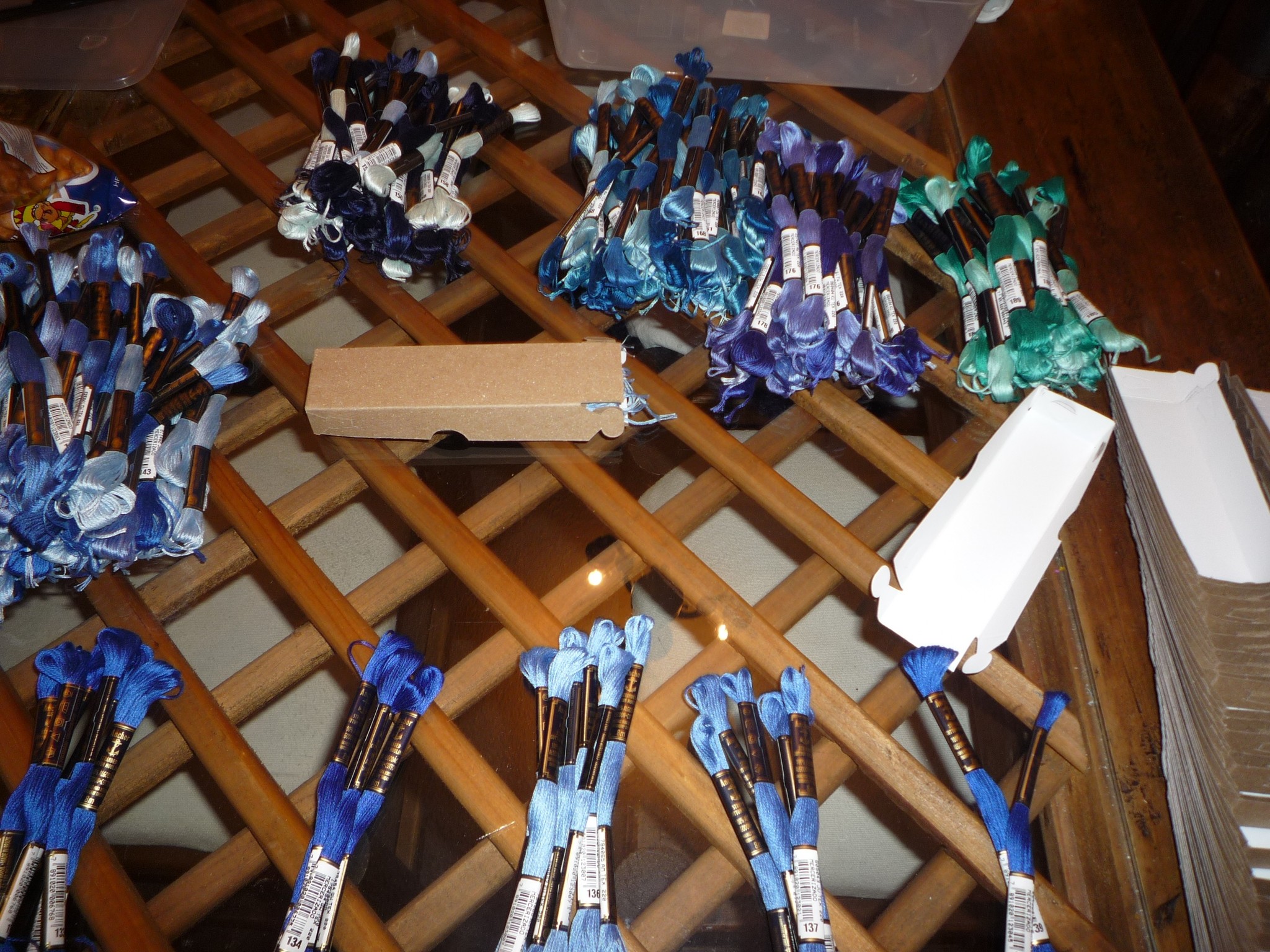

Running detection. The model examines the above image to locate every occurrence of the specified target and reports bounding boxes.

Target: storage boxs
[304,333,630,441]
[543,0,993,93]
